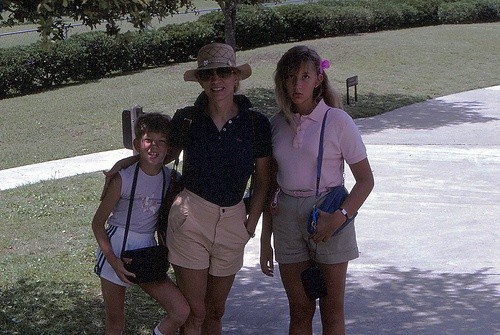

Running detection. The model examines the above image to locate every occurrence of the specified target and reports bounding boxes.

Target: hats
[184,43,252,82]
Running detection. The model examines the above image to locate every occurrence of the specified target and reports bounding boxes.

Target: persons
[92,112,191,335]
[260,45,375,335]
[99,42,270,335]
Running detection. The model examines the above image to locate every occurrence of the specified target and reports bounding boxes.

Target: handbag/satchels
[120,244,171,285]
[156,105,195,230]
[307,107,358,239]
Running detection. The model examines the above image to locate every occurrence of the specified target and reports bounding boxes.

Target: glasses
[194,67,236,79]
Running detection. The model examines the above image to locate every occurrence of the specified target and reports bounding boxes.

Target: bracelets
[339,208,349,220]
[247,229,255,238]
